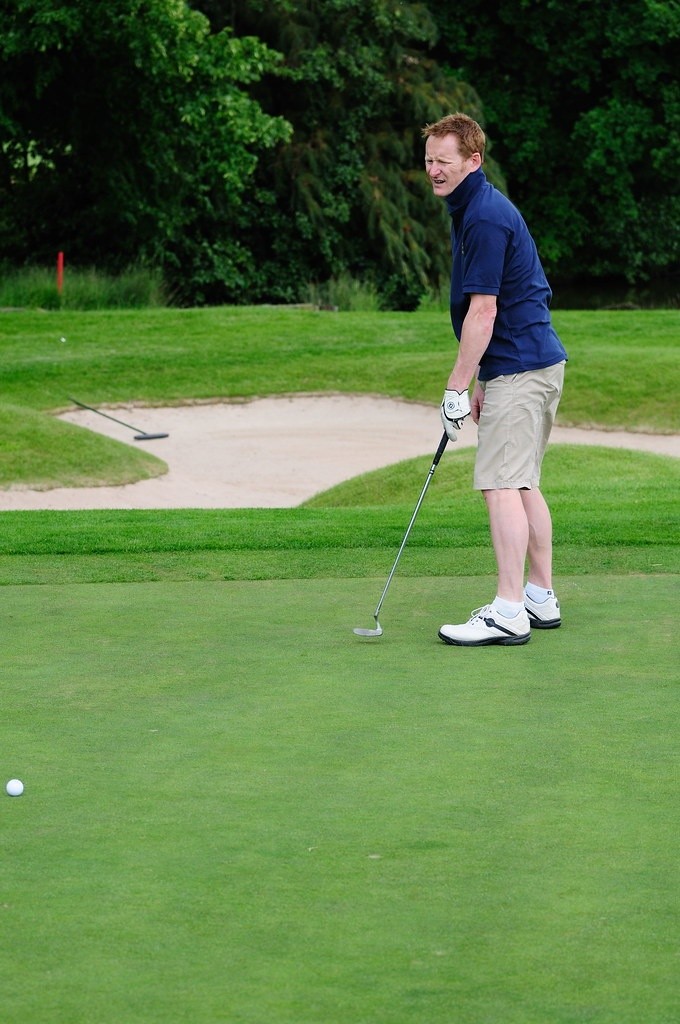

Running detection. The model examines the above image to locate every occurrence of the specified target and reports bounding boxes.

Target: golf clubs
[351,430,449,638]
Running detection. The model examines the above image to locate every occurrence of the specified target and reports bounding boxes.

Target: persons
[423,112,569,646]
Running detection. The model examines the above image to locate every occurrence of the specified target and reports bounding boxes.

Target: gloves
[440,389,471,443]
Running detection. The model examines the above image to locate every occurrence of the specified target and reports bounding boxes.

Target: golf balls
[6,778,23,796]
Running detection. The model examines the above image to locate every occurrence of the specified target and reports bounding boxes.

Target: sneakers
[438,604,531,646]
[525,594,561,628]
